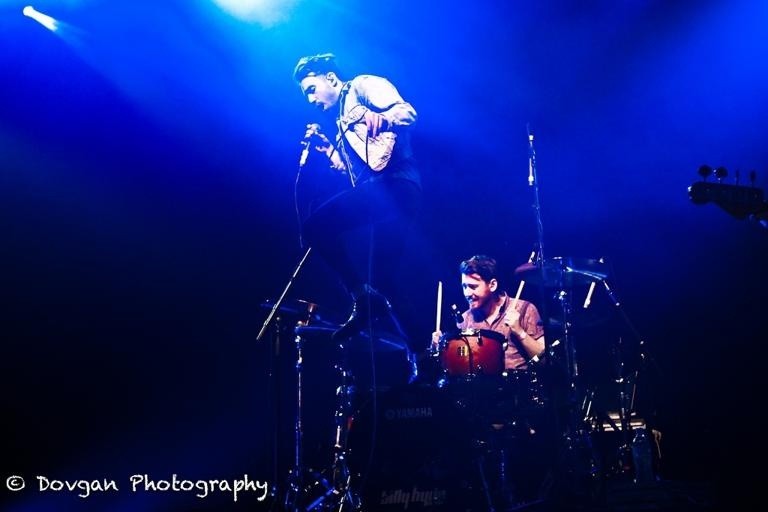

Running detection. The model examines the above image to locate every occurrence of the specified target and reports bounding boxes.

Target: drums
[439,327,509,384]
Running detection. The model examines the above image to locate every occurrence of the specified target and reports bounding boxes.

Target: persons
[291,51,435,345]
[426,252,548,371]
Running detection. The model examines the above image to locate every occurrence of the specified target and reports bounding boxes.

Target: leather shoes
[402,334,441,387]
[327,286,394,342]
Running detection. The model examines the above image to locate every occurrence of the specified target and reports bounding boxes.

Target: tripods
[246,315,352,512]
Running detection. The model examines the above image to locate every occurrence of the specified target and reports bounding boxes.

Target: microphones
[563,262,606,285]
[299,123,322,167]
[527,135,534,186]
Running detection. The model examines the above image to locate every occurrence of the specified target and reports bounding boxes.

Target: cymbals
[515,261,607,289]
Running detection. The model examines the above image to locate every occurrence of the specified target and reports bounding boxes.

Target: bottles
[630,427,654,483]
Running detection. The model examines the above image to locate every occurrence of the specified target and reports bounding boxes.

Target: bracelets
[516,331,529,342]
